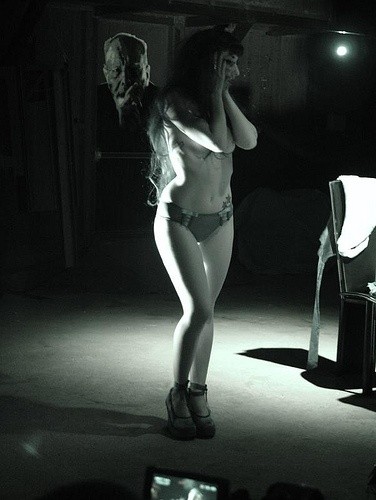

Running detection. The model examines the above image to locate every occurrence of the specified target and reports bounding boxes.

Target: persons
[96,33,158,157]
[154,28,257,441]
[186,487,206,500]
[37,479,140,500]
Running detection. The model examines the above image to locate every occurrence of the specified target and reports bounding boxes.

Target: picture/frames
[92,14,178,159]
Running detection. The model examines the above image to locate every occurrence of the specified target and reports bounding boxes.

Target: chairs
[329,180,376,394]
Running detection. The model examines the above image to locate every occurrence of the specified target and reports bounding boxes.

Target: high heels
[164,380,216,440]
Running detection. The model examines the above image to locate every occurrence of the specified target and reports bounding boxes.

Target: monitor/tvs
[143,466,229,499]
